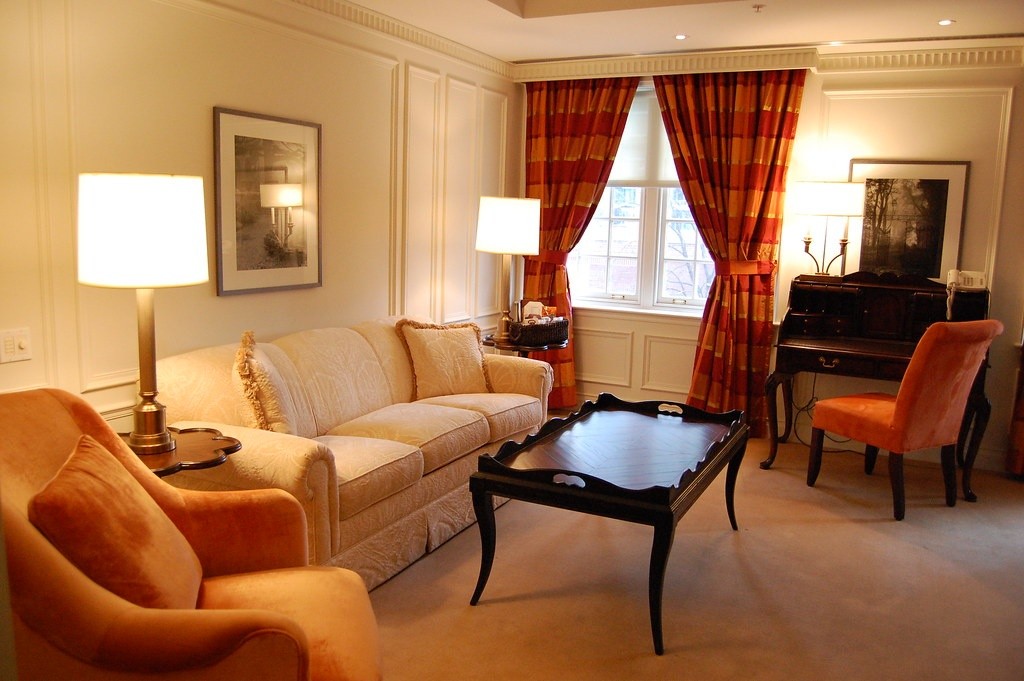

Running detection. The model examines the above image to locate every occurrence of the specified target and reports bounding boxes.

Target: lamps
[260,184,303,250]
[474,195,541,342]
[76,172,209,454]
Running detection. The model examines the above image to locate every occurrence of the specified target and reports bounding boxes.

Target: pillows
[26,433,204,610]
[230,328,297,438]
[394,318,496,402]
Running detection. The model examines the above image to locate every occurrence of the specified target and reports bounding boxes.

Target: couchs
[133,317,556,593]
[0,387,385,681]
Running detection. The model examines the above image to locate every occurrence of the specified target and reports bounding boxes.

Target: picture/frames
[840,158,972,287]
[235,167,288,248]
[213,106,322,297]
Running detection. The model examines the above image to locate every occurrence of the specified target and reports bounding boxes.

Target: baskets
[509,317,568,347]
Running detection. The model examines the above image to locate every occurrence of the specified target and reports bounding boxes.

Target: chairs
[806,319,1003,522]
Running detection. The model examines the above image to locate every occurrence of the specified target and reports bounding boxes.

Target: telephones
[946,270,986,292]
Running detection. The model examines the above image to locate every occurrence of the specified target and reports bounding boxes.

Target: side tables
[116,427,242,479]
[482,337,569,358]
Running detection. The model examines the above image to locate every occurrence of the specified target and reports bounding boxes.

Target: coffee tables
[469,388,750,655]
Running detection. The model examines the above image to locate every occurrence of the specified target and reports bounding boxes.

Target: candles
[288,207,292,222]
[271,208,275,224]
